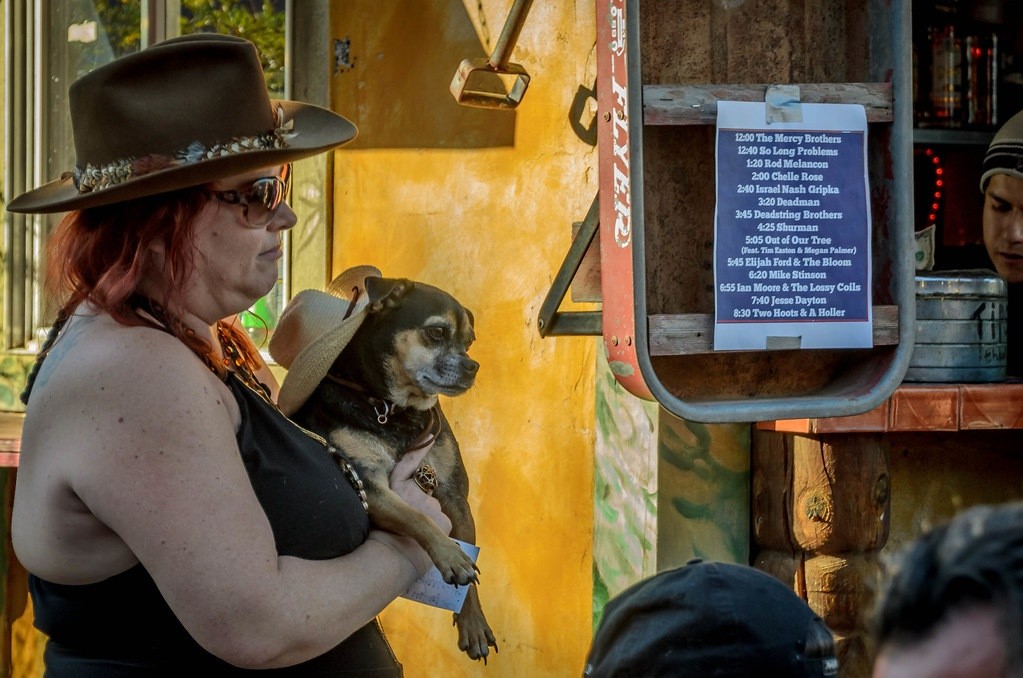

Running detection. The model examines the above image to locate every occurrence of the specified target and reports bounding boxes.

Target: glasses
[206,165,293,229]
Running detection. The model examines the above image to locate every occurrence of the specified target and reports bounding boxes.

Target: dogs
[288,270,502,667]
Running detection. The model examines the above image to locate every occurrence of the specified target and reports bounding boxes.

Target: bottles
[964,19,997,132]
[919,5,964,130]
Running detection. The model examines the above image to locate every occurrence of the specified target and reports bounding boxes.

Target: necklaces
[193,319,368,508]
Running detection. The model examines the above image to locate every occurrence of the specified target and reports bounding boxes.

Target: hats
[269,264,380,416]
[6,33,358,212]
[583,559,838,678]
[980,111,1023,194]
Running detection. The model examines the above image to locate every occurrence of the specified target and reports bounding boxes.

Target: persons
[582,558,839,678]
[864,502,1023,678]
[979,110,1023,381]
[11,33,454,678]
